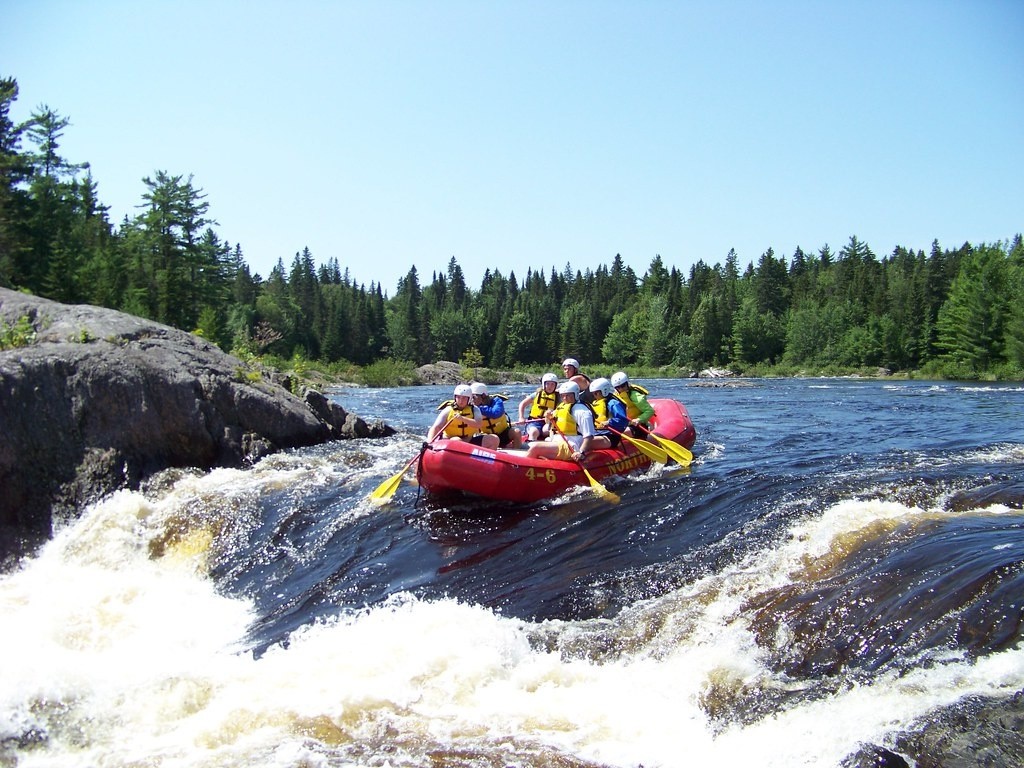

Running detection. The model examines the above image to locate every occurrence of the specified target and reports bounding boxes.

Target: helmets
[541,373,559,389]
[611,372,629,388]
[562,358,579,369]
[589,378,610,396]
[470,383,489,396]
[558,381,579,400]
[454,384,472,405]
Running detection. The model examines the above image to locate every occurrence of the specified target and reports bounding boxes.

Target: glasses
[617,383,627,389]
[593,391,600,395]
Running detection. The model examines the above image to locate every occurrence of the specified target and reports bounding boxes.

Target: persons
[519,373,562,440]
[612,373,654,440]
[470,382,522,449]
[525,358,629,462]
[427,384,499,450]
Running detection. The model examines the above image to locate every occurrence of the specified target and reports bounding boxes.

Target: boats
[413,400,696,498]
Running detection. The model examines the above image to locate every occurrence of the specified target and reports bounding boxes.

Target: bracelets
[580,451,587,456]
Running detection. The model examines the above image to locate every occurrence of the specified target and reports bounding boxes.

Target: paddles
[637,426,695,468]
[549,418,620,504]
[370,410,464,500]
[511,416,545,428]
[595,420,668,464]
